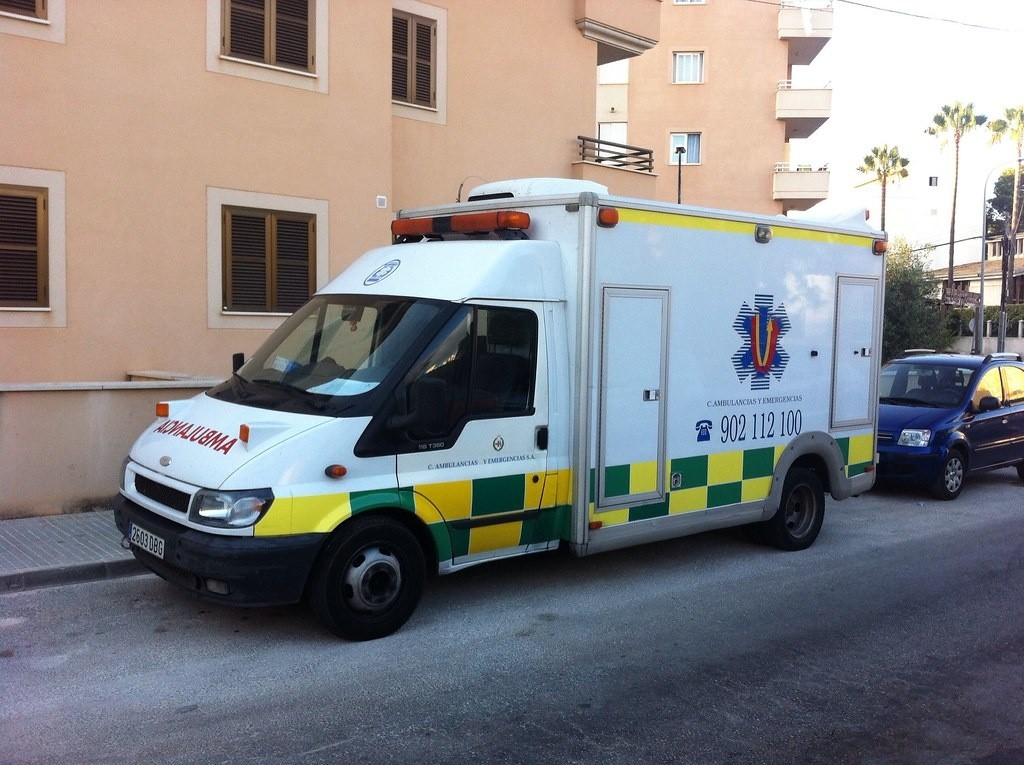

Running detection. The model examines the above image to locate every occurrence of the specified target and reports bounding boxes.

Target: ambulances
[113,178,888,641]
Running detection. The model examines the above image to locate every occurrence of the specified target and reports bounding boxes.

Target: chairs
[906,369,937,402]
[449,313,530,423]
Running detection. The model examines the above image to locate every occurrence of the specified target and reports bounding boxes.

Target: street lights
[975,157,1024,354]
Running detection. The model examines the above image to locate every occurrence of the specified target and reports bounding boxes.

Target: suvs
[877,348,1024,500]
